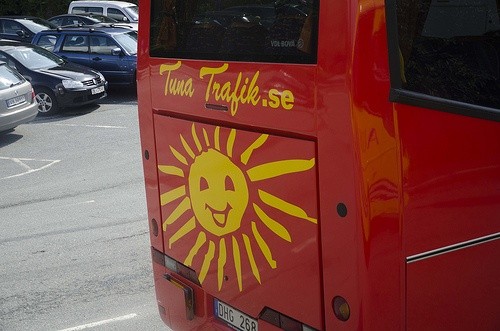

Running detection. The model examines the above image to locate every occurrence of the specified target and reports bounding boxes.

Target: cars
[46,13,138,30]
[0,38,108,131]
[0,15,85,49]
[189,5,308,48]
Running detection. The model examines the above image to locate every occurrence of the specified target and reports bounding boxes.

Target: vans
[67,0,139,23]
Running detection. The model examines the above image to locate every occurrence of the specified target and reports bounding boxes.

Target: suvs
[31,25,138,93]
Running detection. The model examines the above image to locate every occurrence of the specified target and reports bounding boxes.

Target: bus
[138,0,500,331]
